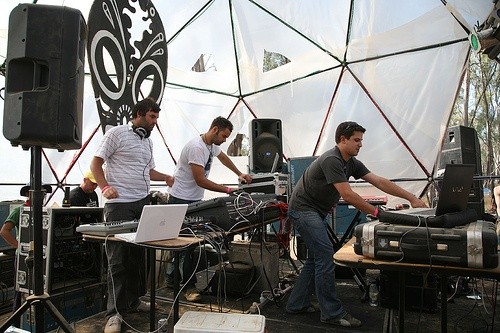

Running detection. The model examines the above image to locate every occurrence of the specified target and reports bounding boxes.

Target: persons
[284,121,429,327]
[0,171,99,329]
[167,116,254,302]
[89,97,174,333]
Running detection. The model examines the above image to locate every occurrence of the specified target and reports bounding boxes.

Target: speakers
[444,124,481,179]
[3,3,89,151]
[250,118,283,173]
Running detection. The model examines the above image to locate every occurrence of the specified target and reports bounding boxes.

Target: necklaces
[205,134,212,155]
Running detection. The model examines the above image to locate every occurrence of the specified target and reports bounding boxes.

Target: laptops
[384,163,477,218]
[113,203,189,245]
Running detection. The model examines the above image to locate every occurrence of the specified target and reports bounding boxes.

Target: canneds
[369,290,379,306]
[158,318,168,333]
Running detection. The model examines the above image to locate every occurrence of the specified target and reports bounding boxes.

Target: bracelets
[102,185,110,192]
[373,209,379,217]
[227,187,231,193]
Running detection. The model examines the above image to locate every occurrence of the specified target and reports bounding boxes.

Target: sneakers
[320,312,362,327]
[183,288,201,302]
[104,315,122,333]
[286,302,320,314]
[137,301,157,313]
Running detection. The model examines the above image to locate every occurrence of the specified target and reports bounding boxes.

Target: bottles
[483,187,491,213]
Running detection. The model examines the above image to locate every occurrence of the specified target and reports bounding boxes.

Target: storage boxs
[174,311,266,333]
[15,207,104,294]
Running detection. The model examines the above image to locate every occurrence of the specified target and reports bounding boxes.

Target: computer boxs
[228,241,280,292]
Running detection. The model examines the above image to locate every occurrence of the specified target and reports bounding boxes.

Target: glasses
[205,153,213,170]
[344,122,357,130]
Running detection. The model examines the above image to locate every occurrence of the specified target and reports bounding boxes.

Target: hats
[84,170,99,186]
[20,182,52,196]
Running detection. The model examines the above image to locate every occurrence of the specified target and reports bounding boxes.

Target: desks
[81,218,288,333]
[332,238,500,333]
